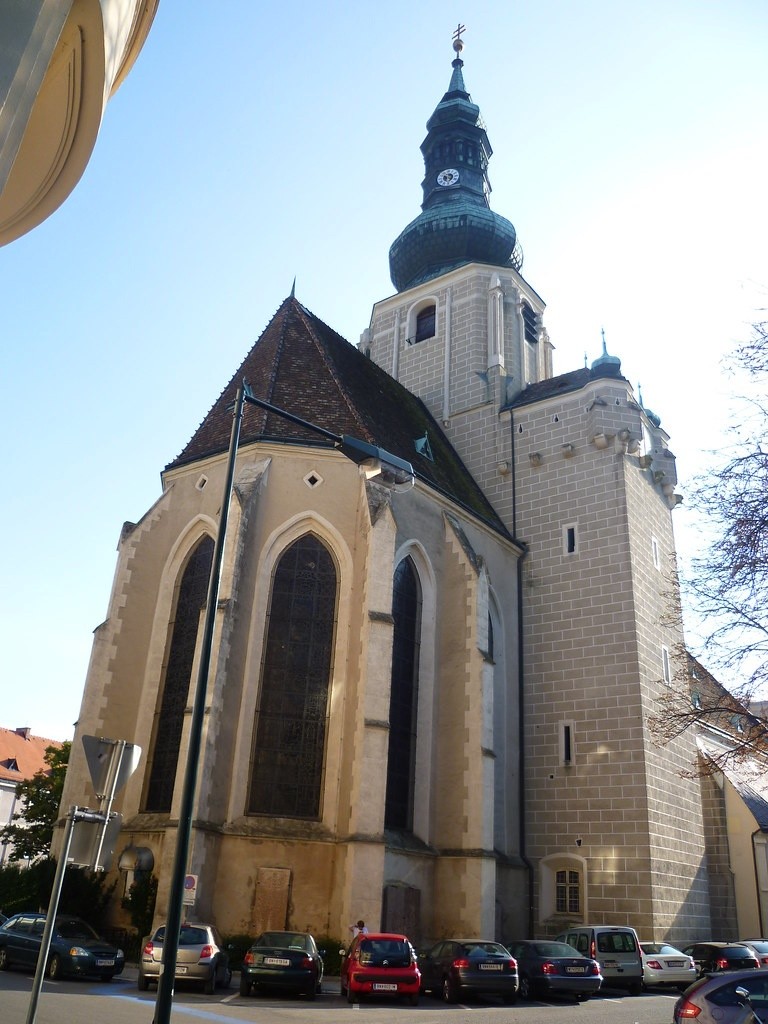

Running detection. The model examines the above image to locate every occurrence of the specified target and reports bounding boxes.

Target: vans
[556,923,644,997]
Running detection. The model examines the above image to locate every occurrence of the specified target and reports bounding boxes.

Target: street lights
[149,387,418,1023]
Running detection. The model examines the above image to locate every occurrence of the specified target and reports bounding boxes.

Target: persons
[349,920,368,939]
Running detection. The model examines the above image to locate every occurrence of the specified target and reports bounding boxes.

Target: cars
[0,911,126,984]
[420,937,521,1008]
[672,968,768,1024]
[735,938,768,971]
[505,940,603,1001]
[336,932,422,1005]
[136,921,233,994]
[239,927,325,1001]
[678,941,761,992]
[637,939,698,993]
